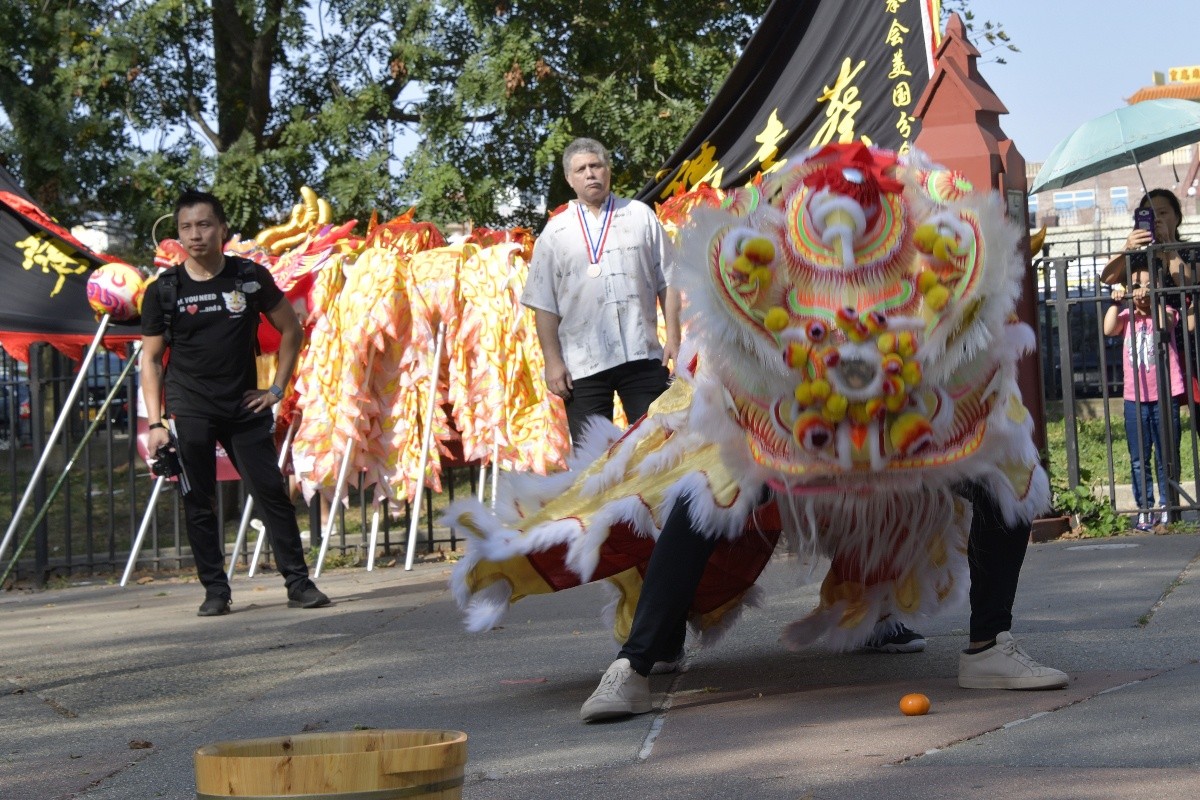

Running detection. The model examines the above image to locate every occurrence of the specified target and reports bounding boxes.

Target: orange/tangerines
[900,693,931,714]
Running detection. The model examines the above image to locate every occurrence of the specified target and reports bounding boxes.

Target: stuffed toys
[439,143,1068,720]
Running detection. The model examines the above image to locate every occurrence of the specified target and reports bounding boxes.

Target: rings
[257,398,262,403]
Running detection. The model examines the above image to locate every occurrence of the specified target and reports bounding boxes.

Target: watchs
[270,385,285,400]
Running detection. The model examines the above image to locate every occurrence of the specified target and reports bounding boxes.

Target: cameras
[153,446,184,478]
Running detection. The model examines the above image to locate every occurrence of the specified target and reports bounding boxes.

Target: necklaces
[188,254,223,279]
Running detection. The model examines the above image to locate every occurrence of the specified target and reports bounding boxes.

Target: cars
[1,341,140,445]
[1038,284,1129,401]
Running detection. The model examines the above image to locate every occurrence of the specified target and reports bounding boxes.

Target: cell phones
[1135,209,1156,243]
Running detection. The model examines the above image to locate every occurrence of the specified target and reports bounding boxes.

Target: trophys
[587,263,602,278]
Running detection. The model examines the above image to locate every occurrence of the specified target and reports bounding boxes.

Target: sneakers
[958,631,1068,689]
[861,624,925,652]
[1136,508,1154,532]
[198,588,233,615]
[287,581,330,608]
[580,659,653,720]
[1159,505,1180,525]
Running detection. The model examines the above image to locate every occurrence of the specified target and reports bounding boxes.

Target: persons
[1101,189,1200,530]
[140,192,331,617]
[520,138,683,462]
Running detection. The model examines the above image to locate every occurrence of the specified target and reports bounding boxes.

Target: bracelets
[149,422,162,430]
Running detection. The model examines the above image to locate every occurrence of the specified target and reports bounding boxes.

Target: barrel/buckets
[196,727,467,800]
